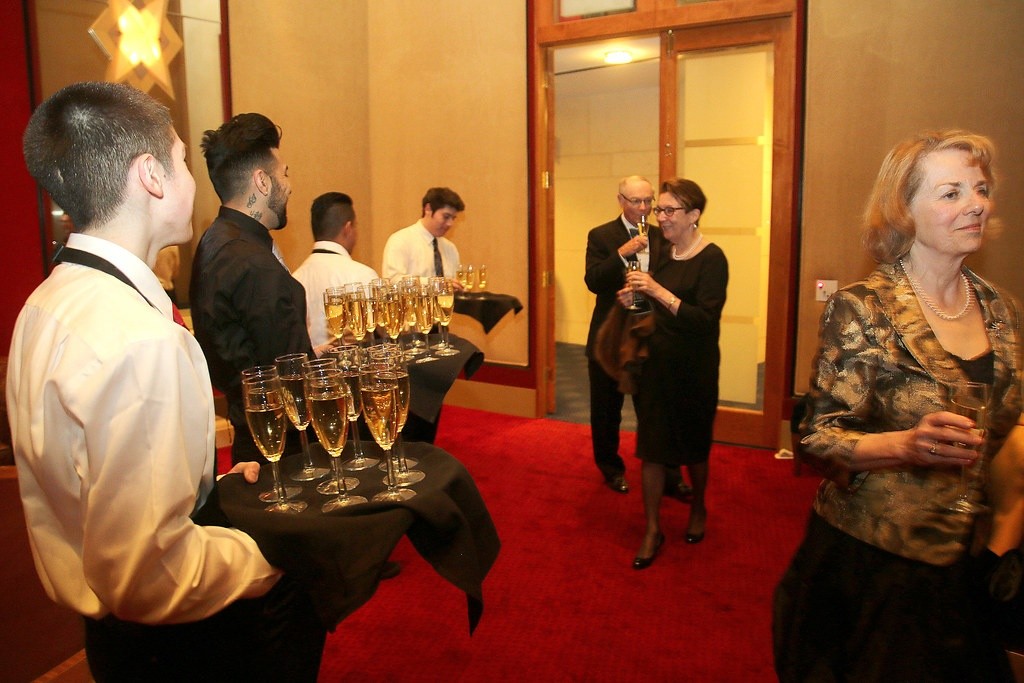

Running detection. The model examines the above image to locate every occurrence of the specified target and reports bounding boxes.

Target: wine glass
[242,278,459,510]
[455,264,487,300]
[625,262,644,310]
[637,215,650,254]
[943,380,994,512]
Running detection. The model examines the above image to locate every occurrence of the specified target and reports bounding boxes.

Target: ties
[271,244,290,273]
[432,238,444,278]
[172,303,191,333]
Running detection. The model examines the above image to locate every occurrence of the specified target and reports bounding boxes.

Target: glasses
[652,205,683,218]
[620,192,653,207]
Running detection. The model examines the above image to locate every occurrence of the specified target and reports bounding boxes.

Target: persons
[5,80,330,683]
[616,178,730,570]
[191,113,338,468]
[584,176,709,502]
[773,129,1024,683]
[291,192,382,345]
[382,188,466,447]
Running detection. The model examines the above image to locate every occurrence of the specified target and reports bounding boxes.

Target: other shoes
[664,477,695,499]
[633,531,665,570]
[683,507,707,545]
[606,474,630,493]
[379,560,402,580]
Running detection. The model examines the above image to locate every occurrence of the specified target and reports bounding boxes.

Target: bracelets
[667,297,677,310]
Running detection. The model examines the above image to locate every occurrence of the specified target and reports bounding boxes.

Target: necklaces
[900,259,976,320]
[672,233,703,260]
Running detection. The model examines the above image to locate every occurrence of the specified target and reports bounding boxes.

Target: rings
[929,442,937,453]
